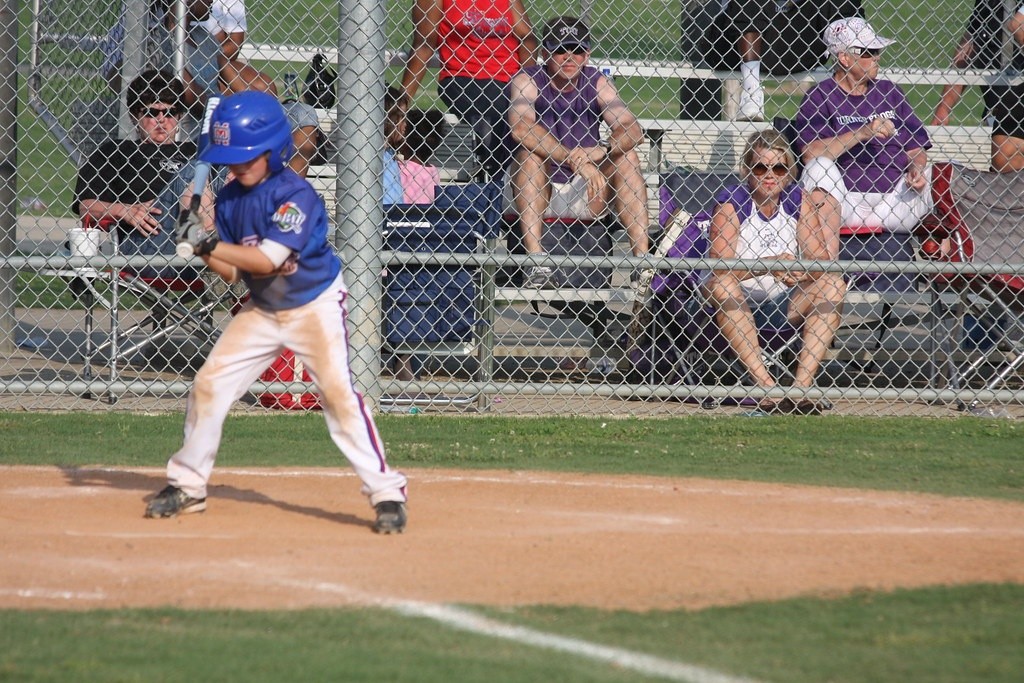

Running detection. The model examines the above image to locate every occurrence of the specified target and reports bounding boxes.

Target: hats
[822,17,897,59]
[543,17,592,50]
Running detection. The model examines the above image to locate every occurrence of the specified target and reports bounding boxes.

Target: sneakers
[736,86,766,122]
[630,254,657,291]
[523,252,560,289]
[374,500,407,534]
[146,485,207,519]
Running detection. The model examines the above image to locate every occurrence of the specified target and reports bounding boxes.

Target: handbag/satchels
[301,53,338,109]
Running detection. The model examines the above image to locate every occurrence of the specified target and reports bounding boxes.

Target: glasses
[848,48,881,58]
[555,43,586,54]
[139,107,181,118]
[751,163,789,177]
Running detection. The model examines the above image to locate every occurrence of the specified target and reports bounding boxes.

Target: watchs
[599,140,611,158]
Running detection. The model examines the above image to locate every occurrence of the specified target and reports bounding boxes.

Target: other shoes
[757,399,792,412]
[795,400,822,415]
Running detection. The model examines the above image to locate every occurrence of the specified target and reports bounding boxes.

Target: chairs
[76,115,1024,413]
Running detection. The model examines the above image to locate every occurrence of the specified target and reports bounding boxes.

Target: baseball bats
[175,93,224,259]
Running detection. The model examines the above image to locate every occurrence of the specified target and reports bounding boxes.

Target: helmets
[197,90,293,173]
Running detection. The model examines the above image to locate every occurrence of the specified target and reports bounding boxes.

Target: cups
[66,228,102,272]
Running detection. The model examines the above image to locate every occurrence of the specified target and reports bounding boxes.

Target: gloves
[173,209,217,256]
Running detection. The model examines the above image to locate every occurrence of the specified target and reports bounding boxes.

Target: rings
[578,157,582,162]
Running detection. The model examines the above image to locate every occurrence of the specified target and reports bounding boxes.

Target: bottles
[602,68,615,86]
[284,73,299,101]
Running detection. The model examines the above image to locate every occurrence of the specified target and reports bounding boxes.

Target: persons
[792,16,963,285]
[400,0,538,181]
[932,0,1024,174]
[146,90,410,536]
[509,15,658,292]
[384,82,409,161]
[698,130,845,414]
[100,0,320,182]
[71,70,238,278]
